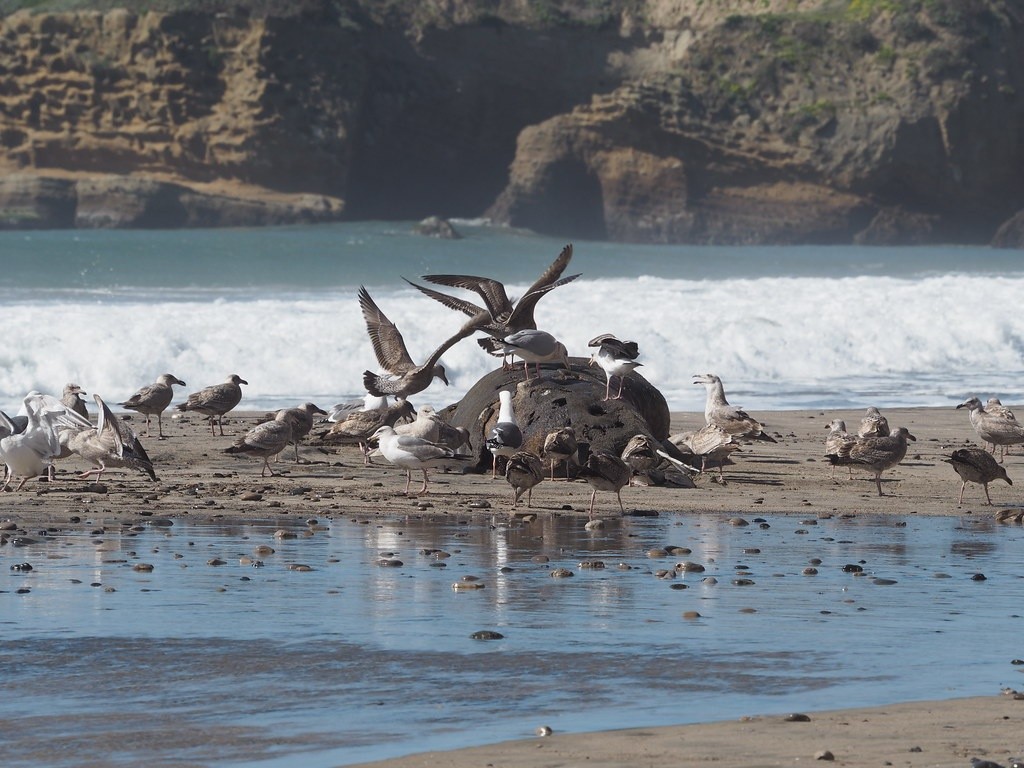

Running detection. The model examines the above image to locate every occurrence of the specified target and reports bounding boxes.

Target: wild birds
[0,241,1023,510]
